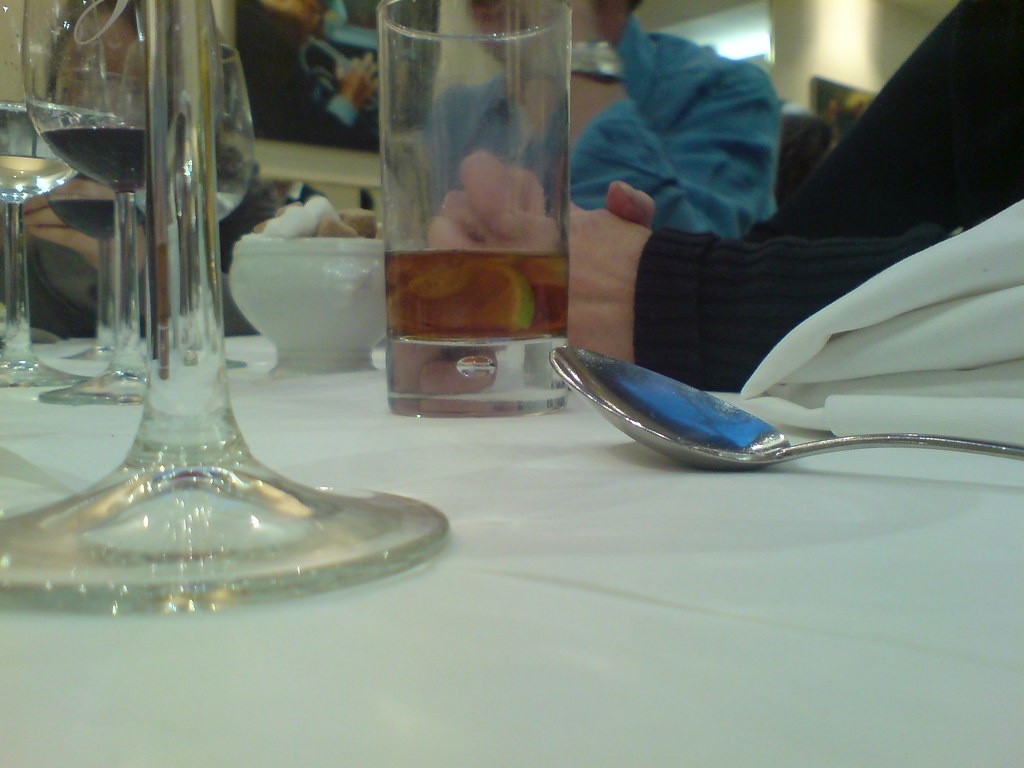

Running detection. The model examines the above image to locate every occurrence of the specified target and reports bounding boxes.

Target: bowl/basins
[229,239,387,378]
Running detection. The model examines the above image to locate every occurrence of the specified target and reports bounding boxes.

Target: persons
[236,0,379,154]
[426,0,1024,394]
[0,0,337,340]
[427,0,782,240]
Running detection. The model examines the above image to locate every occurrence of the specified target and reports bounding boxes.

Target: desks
[0,335,1024,768]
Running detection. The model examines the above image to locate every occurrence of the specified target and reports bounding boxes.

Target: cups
[135,43,259,222]
[376,0,572,418]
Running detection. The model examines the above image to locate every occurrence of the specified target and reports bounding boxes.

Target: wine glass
[0,0,449,612]
[0,0,95,387]
[20,0,151,406]
[44,174,147,362]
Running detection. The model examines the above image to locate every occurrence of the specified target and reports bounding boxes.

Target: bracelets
[532,40,621,81]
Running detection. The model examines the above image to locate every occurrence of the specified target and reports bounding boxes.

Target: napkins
[738,198,1024,449]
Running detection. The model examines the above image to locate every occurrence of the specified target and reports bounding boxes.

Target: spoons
[549,347,1024,471]
